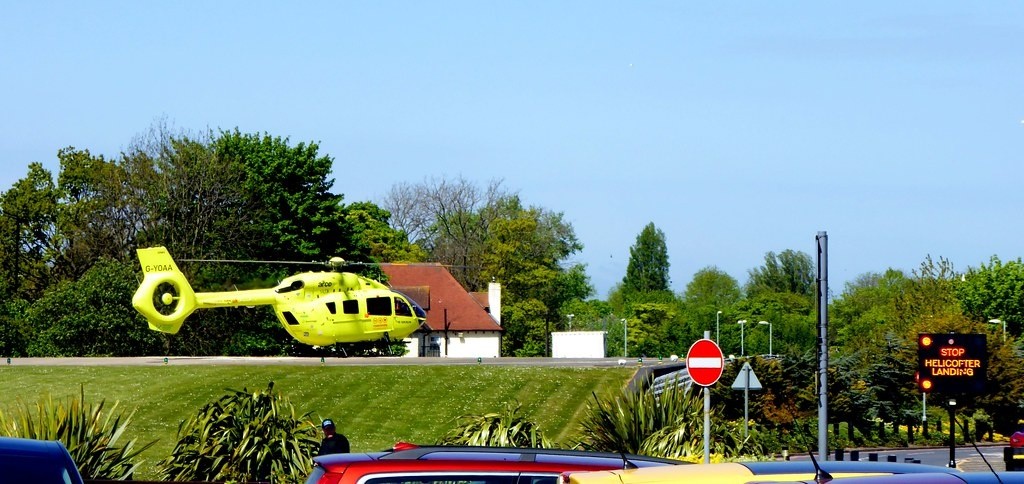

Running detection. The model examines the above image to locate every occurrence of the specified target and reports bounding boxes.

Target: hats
[321,419,334,429]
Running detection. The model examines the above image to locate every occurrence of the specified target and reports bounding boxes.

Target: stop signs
[686,339,729,387]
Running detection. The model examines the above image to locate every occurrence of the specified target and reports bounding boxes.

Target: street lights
[758,320,774,357]
[989,319,1007,345]
[620,319,629,357]
[716,311,725,347]
[737,320,748,356]
[567,313,574,331]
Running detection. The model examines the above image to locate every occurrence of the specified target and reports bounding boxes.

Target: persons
[318,419,350,456]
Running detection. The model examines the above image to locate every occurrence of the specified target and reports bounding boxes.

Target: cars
[1,408,1024,484]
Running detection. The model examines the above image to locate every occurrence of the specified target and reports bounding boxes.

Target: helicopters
[130,244,470,356]
